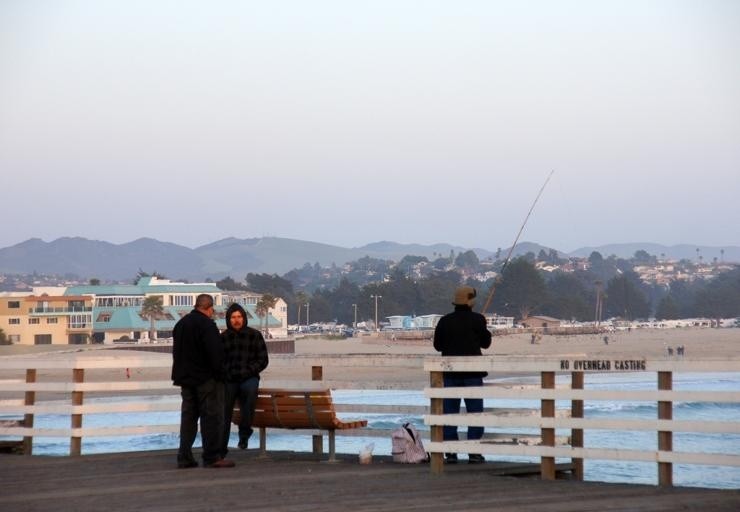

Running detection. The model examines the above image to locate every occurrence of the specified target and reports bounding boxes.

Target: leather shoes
[206,458,234,468]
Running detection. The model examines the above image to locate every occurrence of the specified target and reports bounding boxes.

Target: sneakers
[447,456,457,463]
[469,455,484,463]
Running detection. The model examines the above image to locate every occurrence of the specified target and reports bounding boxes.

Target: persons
[172,293,235,468]
[217,302,268,457]
[432,286,492,464]
[668,343,685,356]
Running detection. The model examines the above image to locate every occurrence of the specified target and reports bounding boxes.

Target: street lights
[370,294,382,330]
[304,302,310,324]
[353,302,361,323]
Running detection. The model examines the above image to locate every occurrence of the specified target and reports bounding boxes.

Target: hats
[451,286,477,306]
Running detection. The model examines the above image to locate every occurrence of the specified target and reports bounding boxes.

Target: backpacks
[392,422,427,464]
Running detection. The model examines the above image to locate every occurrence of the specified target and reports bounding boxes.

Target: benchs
[227,388,368,465]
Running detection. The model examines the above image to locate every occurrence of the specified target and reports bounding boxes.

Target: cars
[288,321,363,337]
[561,317,739,330]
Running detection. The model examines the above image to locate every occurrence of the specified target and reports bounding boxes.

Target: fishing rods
[481,170,553,316]
